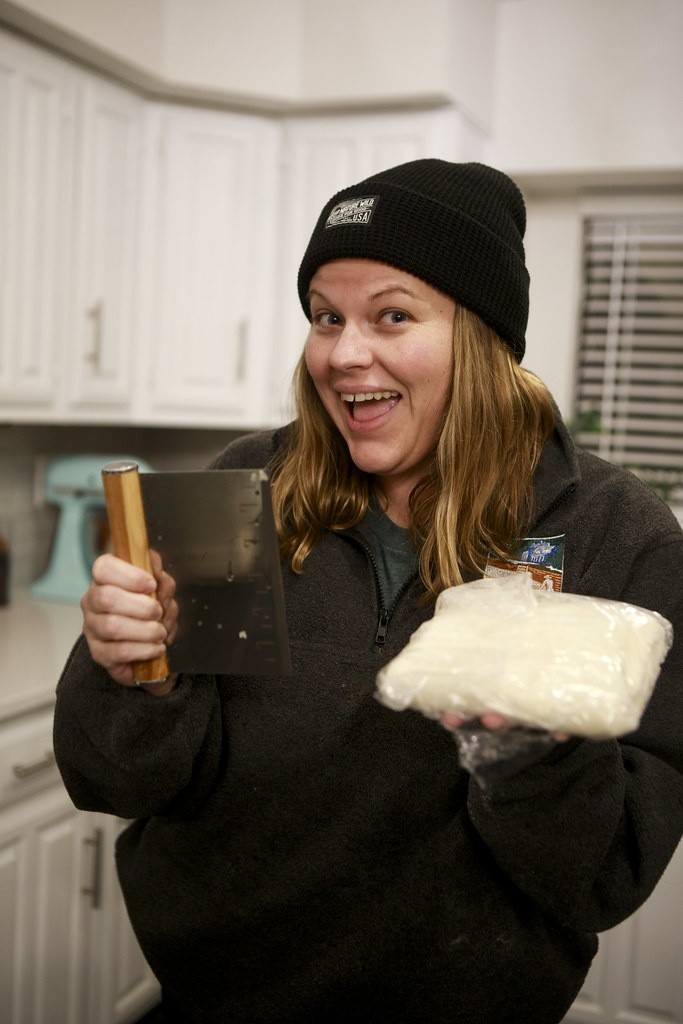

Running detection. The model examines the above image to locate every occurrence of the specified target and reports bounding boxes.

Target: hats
[298,159,530,366]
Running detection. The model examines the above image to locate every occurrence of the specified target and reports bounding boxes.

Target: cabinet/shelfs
[0,703,167,1024]
[0,0,503,433]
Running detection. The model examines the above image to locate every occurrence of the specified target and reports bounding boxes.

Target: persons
[53,157,682,1024]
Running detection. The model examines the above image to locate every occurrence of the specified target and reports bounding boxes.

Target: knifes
[104,459,291,684]
